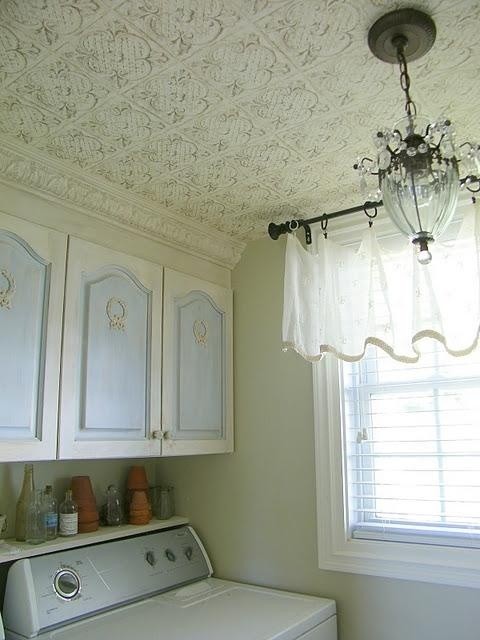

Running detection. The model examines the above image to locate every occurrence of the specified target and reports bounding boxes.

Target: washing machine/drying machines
[3,524,338,640]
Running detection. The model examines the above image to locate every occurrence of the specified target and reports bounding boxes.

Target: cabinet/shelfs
[0,175,75,462]
[62,206,236,460]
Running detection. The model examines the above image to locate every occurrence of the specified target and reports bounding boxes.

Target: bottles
[156,487,174,519]
[26,491,44,544]
[58,488,79,537]
[105,486,122,524]
[44,484,59,540]
[14,464,36,541]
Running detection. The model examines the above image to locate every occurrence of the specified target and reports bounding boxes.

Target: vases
[72,471,101,534]
[126,464,153,525]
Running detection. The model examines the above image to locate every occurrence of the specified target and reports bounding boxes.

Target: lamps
[361,7,478,261]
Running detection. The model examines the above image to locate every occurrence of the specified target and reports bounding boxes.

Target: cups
[124,466,152,526]
[70,474,99,533]
[0,513,8,544]
